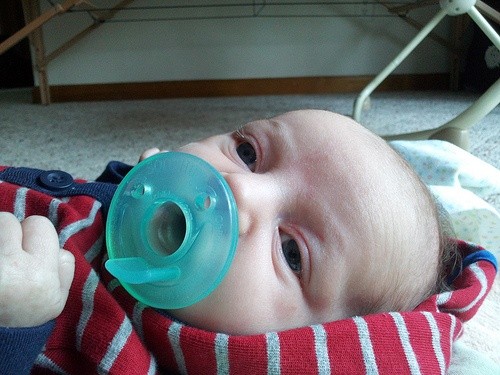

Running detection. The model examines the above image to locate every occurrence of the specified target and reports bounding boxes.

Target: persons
[1,104,498,375]
